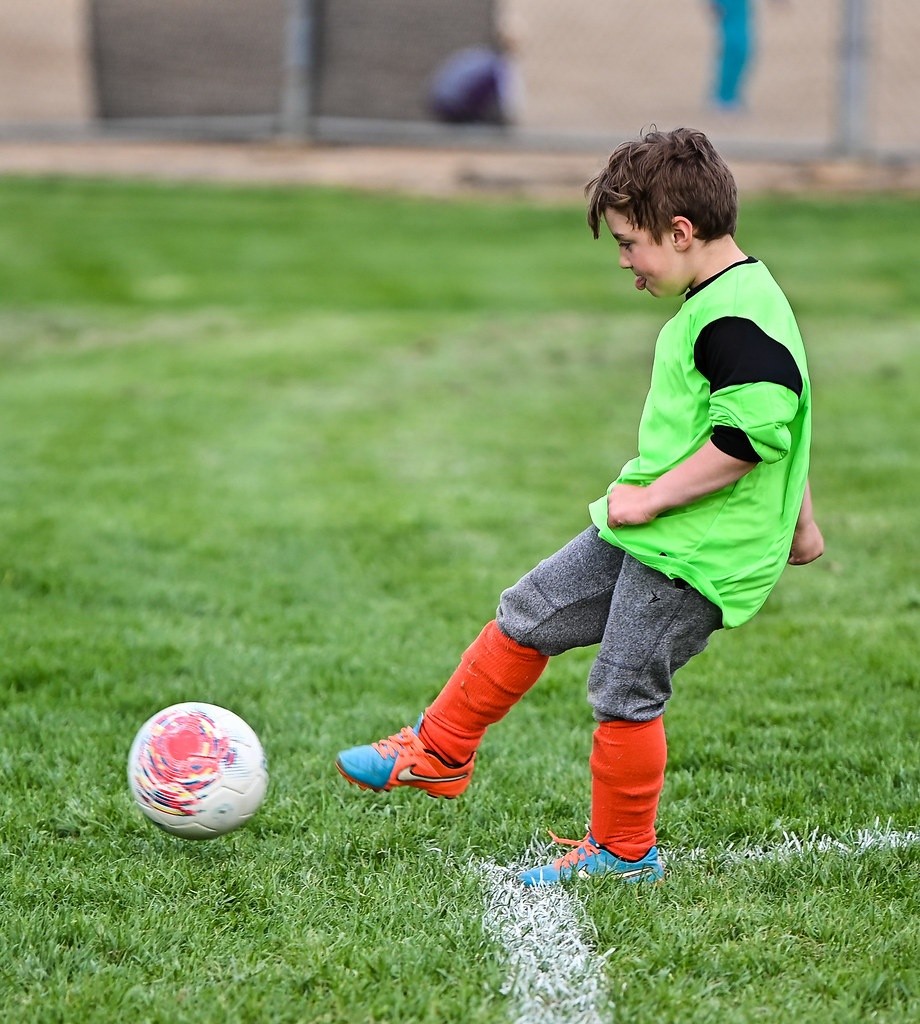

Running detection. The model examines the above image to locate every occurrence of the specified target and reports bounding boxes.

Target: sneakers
[334,711,476,799]
[517,835,664,885]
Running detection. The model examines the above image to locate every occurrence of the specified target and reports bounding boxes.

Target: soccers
[125,702,270,842]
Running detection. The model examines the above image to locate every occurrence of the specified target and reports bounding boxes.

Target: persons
[329,125,824,887]
